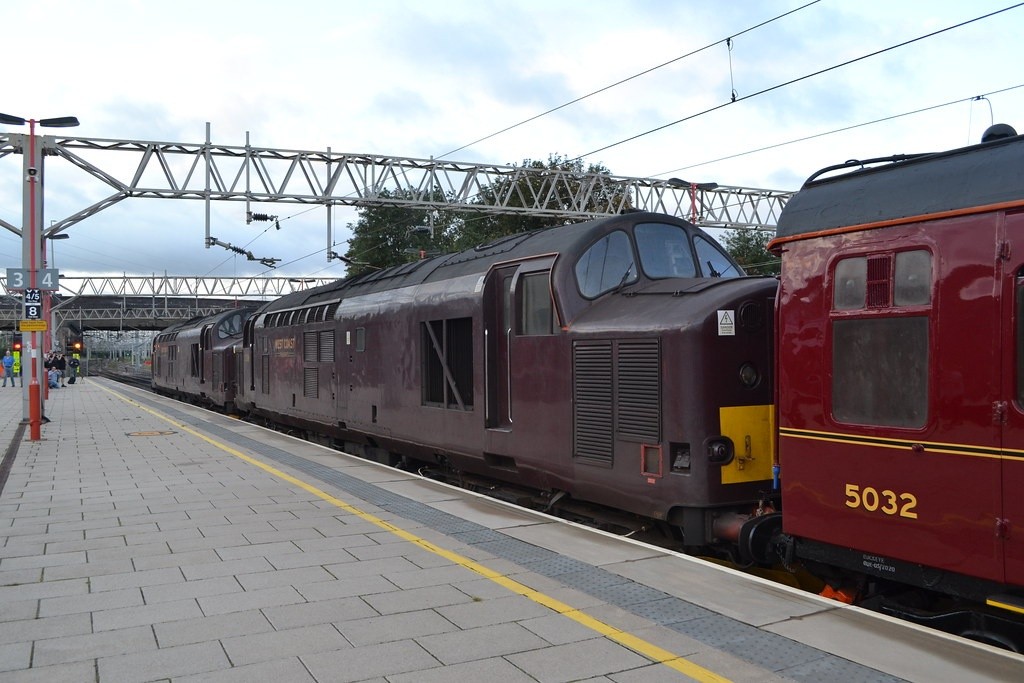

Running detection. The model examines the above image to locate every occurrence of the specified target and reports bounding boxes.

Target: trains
[149,121,1024,658]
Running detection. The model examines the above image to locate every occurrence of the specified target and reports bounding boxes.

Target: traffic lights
[13,332,22,352]
[72,341,81,354]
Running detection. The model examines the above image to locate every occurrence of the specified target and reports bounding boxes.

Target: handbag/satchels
[68,378,74,384]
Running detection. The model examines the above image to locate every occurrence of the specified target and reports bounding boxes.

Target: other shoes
[2,385,5,387]
[11,385,14,387]
[61,385,67,387]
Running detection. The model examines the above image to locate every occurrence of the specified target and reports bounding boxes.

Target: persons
[48,366,62,390]
[68,356,81,386]
[44,350,67,388]
[1,351,15,387]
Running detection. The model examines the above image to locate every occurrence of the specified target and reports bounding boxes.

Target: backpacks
[45,360,50,368]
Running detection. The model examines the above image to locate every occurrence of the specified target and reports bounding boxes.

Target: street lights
[403,248,442,260]
[289,277,317,291]
[667,177,719,226]
[0,112,81,442]
[50,219,59,355]
[42,233,71,400]
